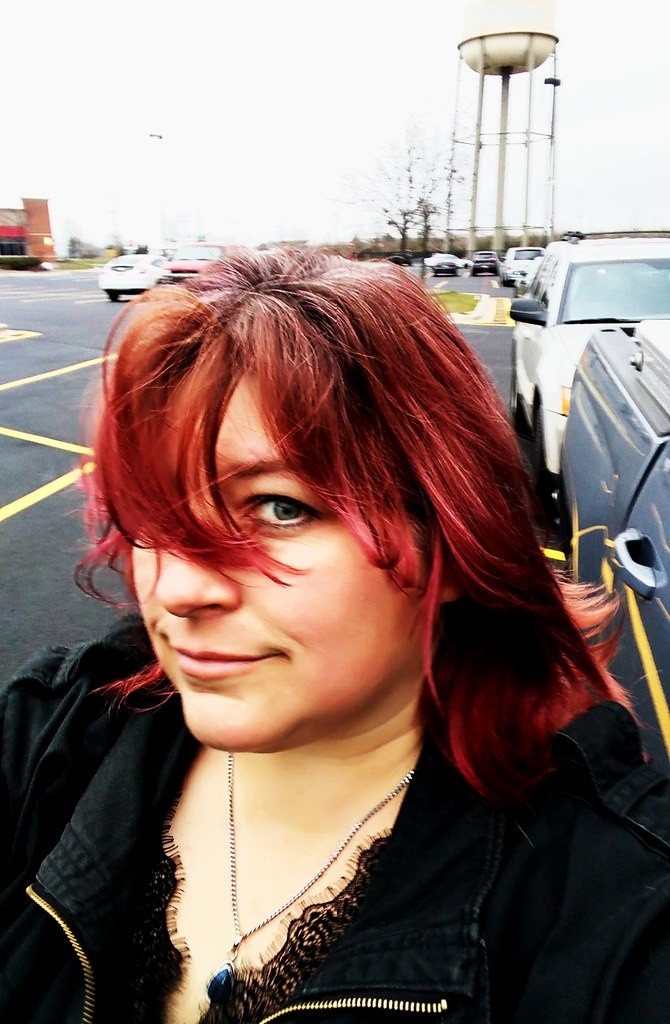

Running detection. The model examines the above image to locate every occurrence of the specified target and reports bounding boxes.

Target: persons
[0,245,670,1024]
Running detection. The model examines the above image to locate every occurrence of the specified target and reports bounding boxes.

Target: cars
[424,254,473,269]
[387,256,411,266]
[433,260,458,276]
[472,251,499,276]
[560,320,670,777]
[498,247,546,287]
[99,254,171,301]
[511,257,543,292]
[156,242,247,285]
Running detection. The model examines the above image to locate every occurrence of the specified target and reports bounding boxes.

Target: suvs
[510,231,670,528]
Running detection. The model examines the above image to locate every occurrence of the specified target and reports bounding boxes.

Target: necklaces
[206,752,416,1011]
